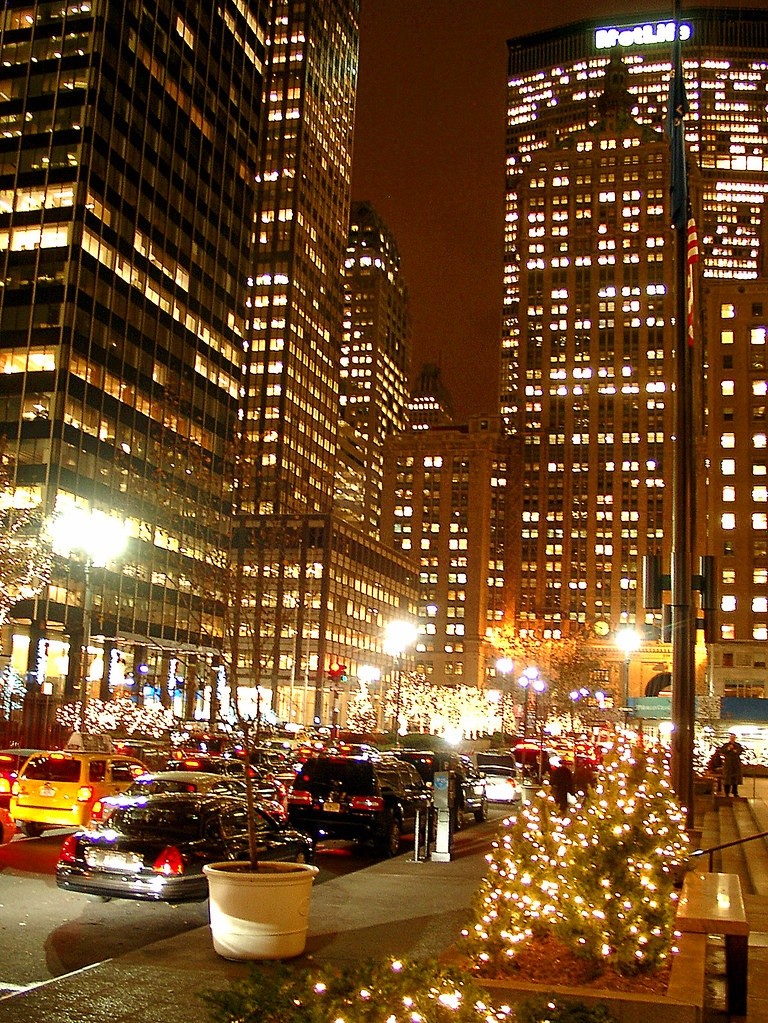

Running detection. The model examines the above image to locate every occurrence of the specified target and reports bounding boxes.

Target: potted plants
[87,378,319,960]
[483,649,601,807]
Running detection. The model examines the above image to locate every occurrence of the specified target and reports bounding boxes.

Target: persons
[549,760,575,811]
[709,747,723,771]
[721,733,744,797]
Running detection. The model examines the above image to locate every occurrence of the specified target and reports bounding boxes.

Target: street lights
[389,619,416,746]
[498,657,513,748]
[63,505,119,746]
[519,668,538,740]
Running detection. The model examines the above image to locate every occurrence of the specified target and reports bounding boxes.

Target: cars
[0,750,26,845]
[56,791,314,904]
[477,765,525,805]
[116,771,287,827]
[102,728,620,816]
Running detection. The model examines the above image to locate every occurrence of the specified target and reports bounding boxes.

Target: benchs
[676,872,750,1015]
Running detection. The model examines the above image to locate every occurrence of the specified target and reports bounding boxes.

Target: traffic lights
[332,664,340,681]
[328,664,335,680]
[339,664,347,681]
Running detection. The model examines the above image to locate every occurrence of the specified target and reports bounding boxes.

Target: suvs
[285,753,435,861]
[394,751,488,832]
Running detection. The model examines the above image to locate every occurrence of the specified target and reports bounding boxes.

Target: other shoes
[734,794,739,798]
[725,795,729,798]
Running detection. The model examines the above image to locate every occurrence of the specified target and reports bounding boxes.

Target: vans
[9,750,154,837]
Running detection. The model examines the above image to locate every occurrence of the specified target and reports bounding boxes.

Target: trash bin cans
[431,771,458,862]
